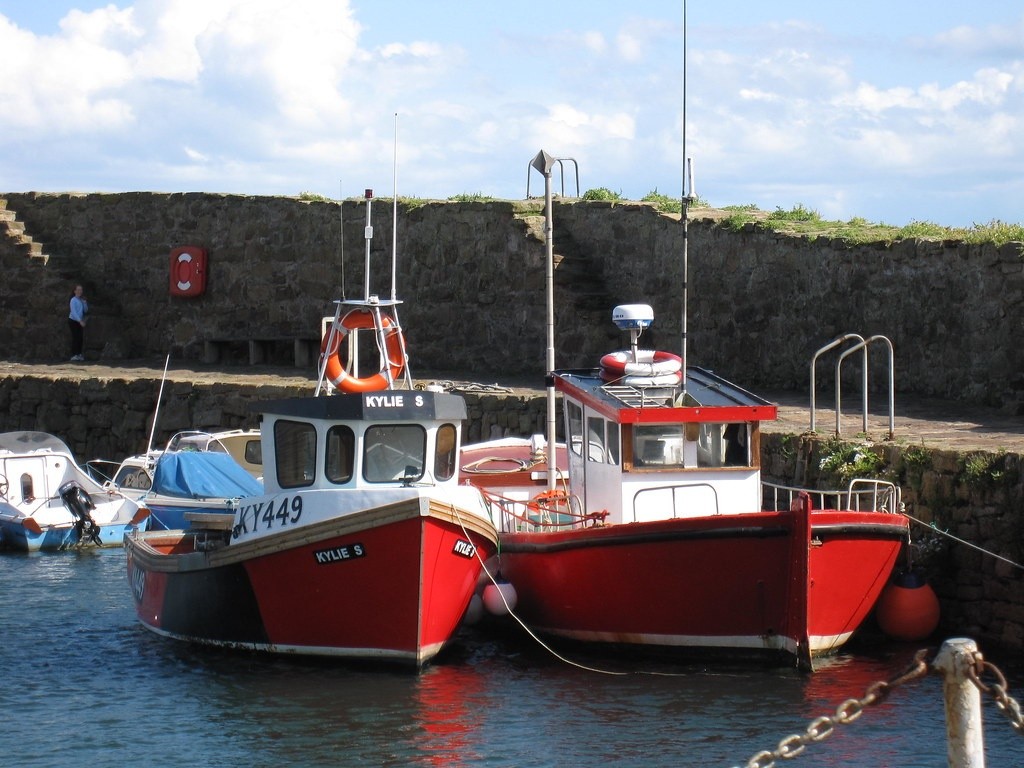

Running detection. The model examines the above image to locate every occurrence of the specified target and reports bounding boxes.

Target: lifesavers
[594,348,688,392]
[315,304,411,393]
[521,486,572,533]
[173,252,197,290]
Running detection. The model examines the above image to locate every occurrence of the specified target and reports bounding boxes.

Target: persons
[67,281,92,361]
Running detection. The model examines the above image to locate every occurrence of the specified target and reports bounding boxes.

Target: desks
[524,514,573,533]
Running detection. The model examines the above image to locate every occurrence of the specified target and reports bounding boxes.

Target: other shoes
[70,355,78,361]
[78,355,85,361]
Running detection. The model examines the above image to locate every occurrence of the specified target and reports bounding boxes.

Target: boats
[0,430,154,557]
[489,0,916,673]
[86,426,346,536]
[121,108,508,670]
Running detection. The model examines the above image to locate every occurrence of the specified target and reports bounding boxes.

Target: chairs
[642,439,666,463]
[487,492,530,532]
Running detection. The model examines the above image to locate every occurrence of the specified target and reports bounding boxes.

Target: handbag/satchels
[84,314,89,323]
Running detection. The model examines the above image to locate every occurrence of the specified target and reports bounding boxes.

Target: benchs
[200,334,320,367]
